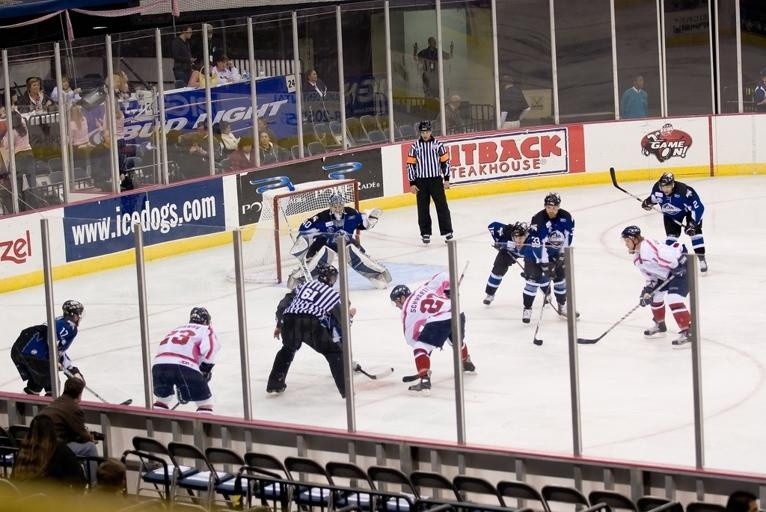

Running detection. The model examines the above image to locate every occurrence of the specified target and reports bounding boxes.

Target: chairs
[0,425,32,468]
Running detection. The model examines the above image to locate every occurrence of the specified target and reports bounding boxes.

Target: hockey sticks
[577,273,676,345]
[533,259,555,345]
[403,261,470,382]
[58,362,132,405]
[506,252,580,318]
[358,367,394,379]
[610,168,688,228]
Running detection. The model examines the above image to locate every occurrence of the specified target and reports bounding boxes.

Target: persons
[151,306,216,416]
[412,35,453,118]
[642,172,708,273]
[265,263,356,399]
[725,491,758,512]
[499,73,531,130]
[407,120,455,246]
[390,271,474,391]
[1,24,328,213]
[754,67,766,112]
[483,221,551,306]
[9,415,86,499]
[433,94,463,135]
[527,192,574,247]
[86,459,126,501]
[521,230,580,323]
[621,226,700,348]
[36,377,98,491]
[11,301,84,396]
[287,191,390,291]
[619,74,649,119]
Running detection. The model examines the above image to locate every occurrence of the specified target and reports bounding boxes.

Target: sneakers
[699,257,707,272]
[464,361,474,371]
[644,321,667,335]
[522,308,532,323]
[672,329,692,345]
[484,295,494,304]
[408,376,431,391]
[559,310,580,318]
[267,384,286,392]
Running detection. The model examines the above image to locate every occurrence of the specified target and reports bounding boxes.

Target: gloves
[641,195,657,210]
[684,221,697,235]
[672,255,687,277]
[639,286,654,306]
[540,262,557,279]
[553,253,565,275]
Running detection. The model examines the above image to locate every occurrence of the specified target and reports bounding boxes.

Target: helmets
[190,306,211,324]
[510,221,528,236]
[545,191,561,205]
[390,284,411,301]
[659,172,674,186]
[418,120,432,131]
[62,300,84,315]
[328,192,347,221]
[317,263,338,287]
[622,225,640,238]
